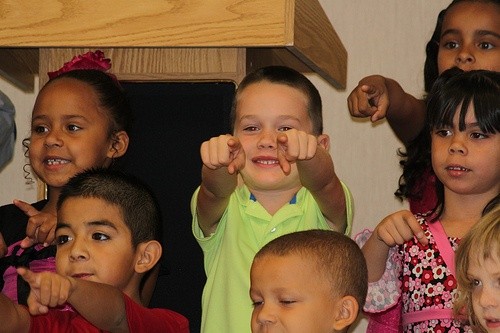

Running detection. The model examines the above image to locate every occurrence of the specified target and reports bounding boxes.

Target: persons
[0,50,190,333]
[190,66,355,333]
[348,1,499,332]
[249,229,369,333]
